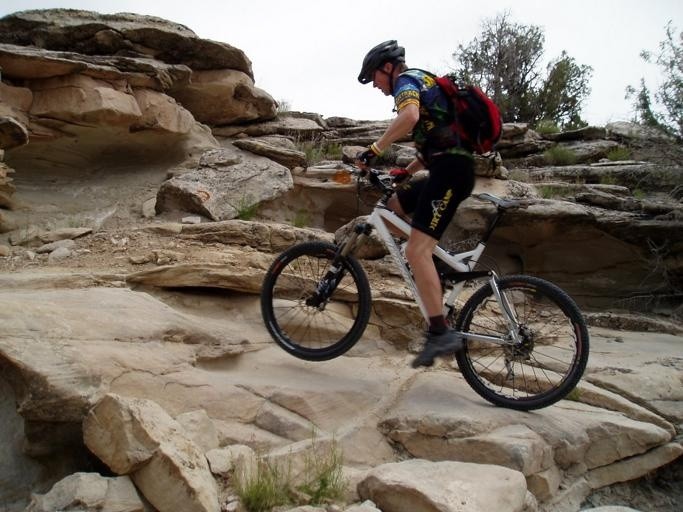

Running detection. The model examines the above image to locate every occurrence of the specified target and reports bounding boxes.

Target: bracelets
[371,142,384,158]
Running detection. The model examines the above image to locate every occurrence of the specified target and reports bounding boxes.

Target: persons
[355,39,474,368]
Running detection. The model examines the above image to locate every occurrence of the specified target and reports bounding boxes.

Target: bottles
[398,235,417,283]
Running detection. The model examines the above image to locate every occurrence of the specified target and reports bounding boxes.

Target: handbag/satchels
[412,67,503,155]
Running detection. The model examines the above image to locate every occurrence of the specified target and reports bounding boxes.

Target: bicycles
[257,147,593,416]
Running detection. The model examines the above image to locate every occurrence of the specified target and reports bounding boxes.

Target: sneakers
[413,333,462,368]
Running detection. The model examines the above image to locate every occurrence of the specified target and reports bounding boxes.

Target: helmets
[358,40,405,84]
